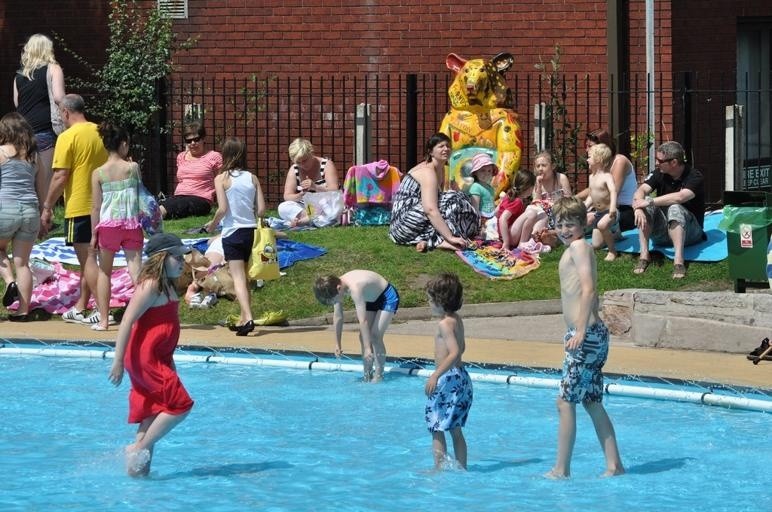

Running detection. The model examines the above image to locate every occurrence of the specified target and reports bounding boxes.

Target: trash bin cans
[727,201,772,293]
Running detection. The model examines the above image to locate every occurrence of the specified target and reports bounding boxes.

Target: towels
[23,234,211,264]
[195,234,327,270]
[6,263,141,314]
[460,243,542,283]
[342,157,405,209]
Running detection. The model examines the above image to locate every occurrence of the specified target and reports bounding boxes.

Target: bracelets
[43,202,51,209]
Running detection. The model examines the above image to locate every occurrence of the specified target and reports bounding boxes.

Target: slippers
[672,263,685,279]
[633,259,649,274]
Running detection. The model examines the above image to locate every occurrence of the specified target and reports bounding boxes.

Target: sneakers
[189,293,217,309]
[62,307,114,331]
[254,309,287,325]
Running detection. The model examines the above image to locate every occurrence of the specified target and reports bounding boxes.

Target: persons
[204,137,266,336]
[40,94,116,326]
[417,273,473,473]
[313,269,399,383]
[278,138,344,228]
[0,112,51,322]
[107,233,194,478]
[159,121,224,220]
[90,121,144,331]
[388,129,708,280]
[13,34,65,229]
[542,198,625,480]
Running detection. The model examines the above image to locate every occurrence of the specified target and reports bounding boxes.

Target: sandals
[519,237,551,253]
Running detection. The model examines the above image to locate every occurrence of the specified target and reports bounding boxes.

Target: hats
[469,154,498,177]
[145,233,191,255]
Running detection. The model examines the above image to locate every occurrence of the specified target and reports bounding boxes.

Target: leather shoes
[3,282,17,306]
[229,321,254,336]
[8,314,27,321]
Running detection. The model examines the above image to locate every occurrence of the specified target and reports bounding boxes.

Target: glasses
[185,137,201,143]
[657,158,676,163]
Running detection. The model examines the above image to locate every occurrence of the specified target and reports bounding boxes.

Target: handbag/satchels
[138,181,164,236]
[247,227,280,280]
[49,90,66,135]
